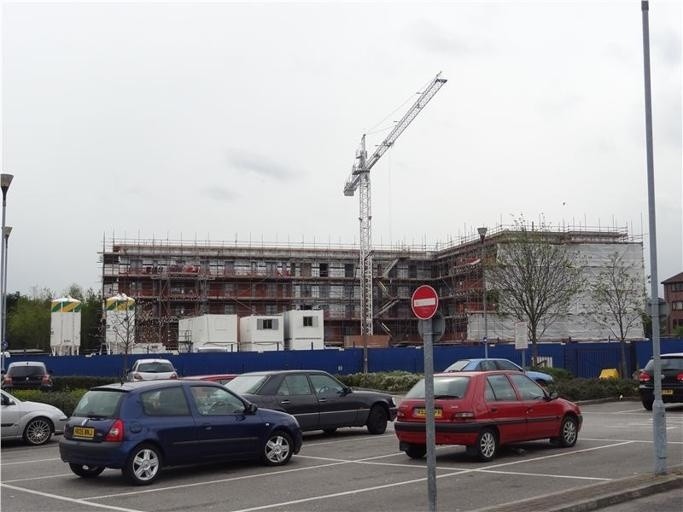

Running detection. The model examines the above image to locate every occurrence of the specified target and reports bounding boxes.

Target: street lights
[0,170,16,379]
[476,226,491,359]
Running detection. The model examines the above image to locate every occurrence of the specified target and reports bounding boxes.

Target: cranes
[341,70,452,337]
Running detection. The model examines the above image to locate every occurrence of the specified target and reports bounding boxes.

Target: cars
[439,357,556,391]
[0,361,56,391]
[0,388,67,446]
[393,370,583,462]
[122,358,178,383]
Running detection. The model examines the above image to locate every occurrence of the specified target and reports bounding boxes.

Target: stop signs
[410,286,439,319]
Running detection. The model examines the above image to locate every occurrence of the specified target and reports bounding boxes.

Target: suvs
[638,351,682,411]
